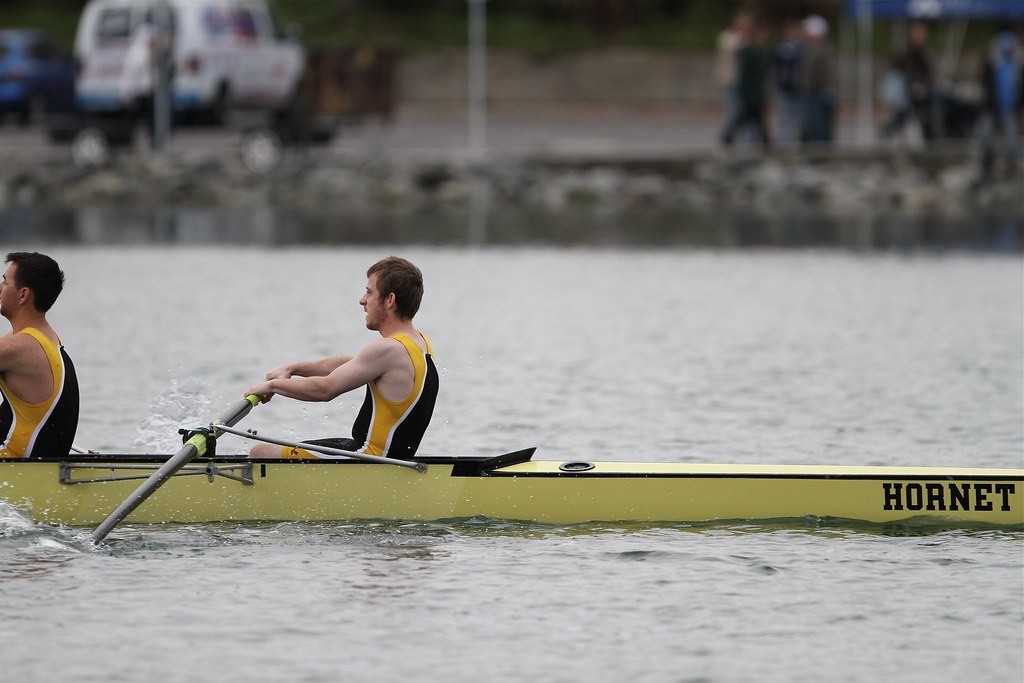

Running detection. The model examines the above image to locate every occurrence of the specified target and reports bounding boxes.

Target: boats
[1,450,1024,527]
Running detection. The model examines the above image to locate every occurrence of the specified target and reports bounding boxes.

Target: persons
[243,256,439,461]
[713,9,1024,148]
[0,253,80,455]
